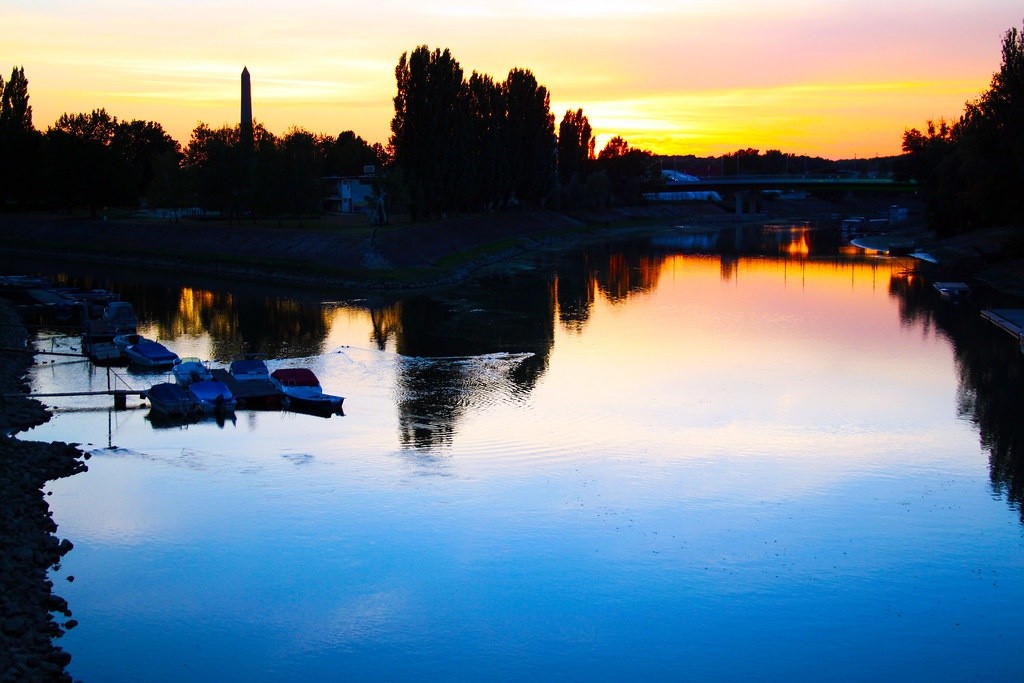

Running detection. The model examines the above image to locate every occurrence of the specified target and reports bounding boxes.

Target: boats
[53,286,139,334]
[273,368,343,410]
[227,360,280,405]
[144,384,203,420]
[173,359,240,414]
[111,334,181,373]
